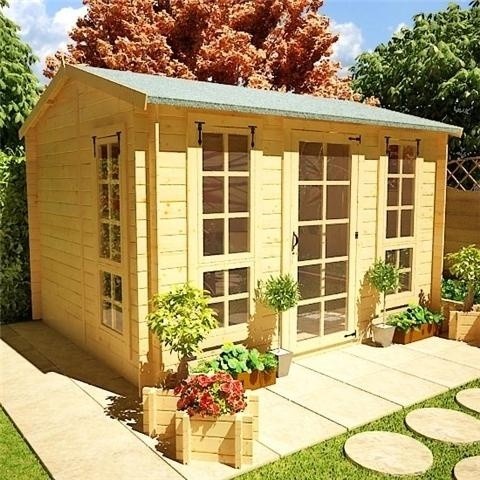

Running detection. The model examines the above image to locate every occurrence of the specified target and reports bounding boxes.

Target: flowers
[172,369,246,418]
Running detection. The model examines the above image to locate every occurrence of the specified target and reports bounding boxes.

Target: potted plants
[264,273,304,378]
[446,242,480,341]
[386,303,442,346]
[205,337,277,391]
[140,279,217,438]
[364,258,397,347]
